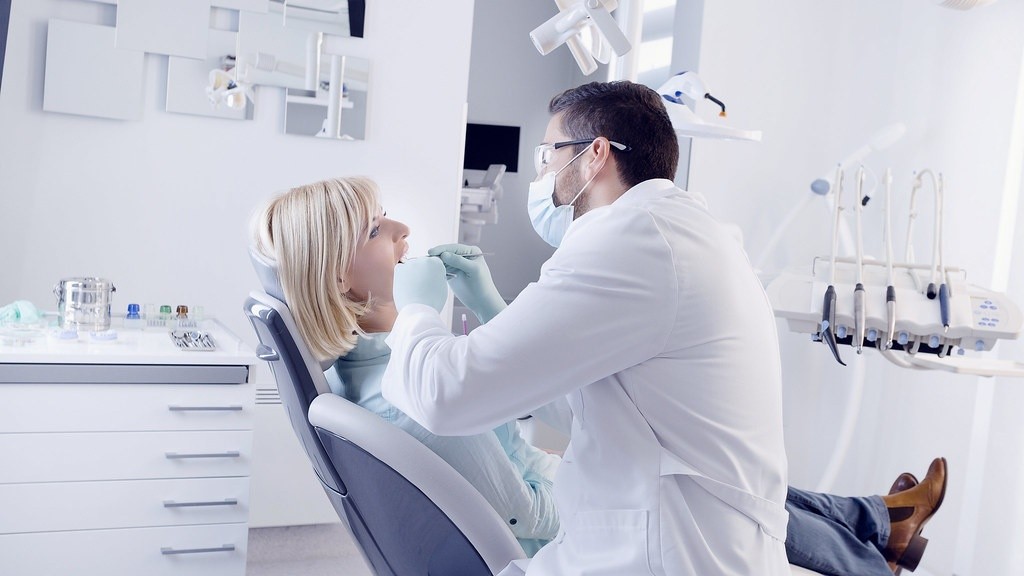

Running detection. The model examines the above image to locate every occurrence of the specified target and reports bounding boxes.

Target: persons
[247,176,947,576]
[380,80,793,576]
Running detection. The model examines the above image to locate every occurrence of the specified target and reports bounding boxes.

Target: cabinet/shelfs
[0,317,257,576]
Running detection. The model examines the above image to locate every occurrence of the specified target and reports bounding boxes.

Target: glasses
[533,138,627,176]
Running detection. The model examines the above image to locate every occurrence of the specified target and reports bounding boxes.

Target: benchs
[243,235,528,575]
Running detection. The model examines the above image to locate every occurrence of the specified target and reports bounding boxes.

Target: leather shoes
[882,457,948,576]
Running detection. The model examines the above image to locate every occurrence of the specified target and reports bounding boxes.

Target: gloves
[428,243,508,326]
[392,256,448,314]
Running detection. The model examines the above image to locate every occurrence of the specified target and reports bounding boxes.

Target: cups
[53,278,117,332]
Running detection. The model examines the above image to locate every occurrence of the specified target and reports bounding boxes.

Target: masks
[527,141,606,248]
